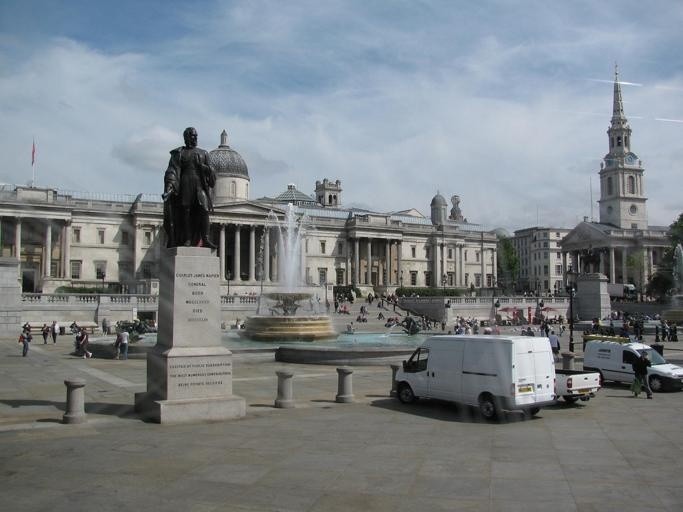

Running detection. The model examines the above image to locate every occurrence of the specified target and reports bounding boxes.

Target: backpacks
[629,377,642,395]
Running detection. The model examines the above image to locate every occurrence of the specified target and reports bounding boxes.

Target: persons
[631,351,652,399]
[160,126,219,250]
[17,318,156,361]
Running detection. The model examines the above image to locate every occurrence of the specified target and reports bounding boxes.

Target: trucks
[606,282,636,301]
[582,339,683,392]
[394,335,556,421]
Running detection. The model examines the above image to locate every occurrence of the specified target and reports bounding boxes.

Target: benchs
[28,325,50,334]
[78,325,100,334]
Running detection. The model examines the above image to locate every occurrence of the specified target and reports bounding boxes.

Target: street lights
[513,279,517,298]
[441,273,447,296]
[491,275,496,297]
[399,275,403,297]
[535,277,540,297]
[101,271,104,294]
[259,270,265,294]
[564,262,579,353]
[553,280,558,297]
[225,270,230,296]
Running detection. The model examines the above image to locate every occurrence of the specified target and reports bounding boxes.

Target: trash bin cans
[650,344,663,357]
[60,327,65,335]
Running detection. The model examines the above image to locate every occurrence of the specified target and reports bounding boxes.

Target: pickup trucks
[547,368,601,407]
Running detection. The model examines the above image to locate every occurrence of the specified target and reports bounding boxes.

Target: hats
[642,350,649,355]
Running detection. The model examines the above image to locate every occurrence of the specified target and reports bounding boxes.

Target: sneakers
[88,352,93,358]
[81,356,87,359]
